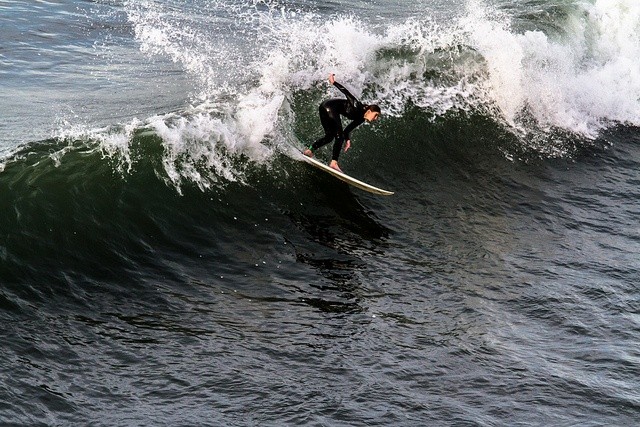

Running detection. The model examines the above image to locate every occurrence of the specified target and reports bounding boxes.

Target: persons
[303,72,381,172]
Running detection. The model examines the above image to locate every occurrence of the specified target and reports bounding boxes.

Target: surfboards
[284,133,395,196]
